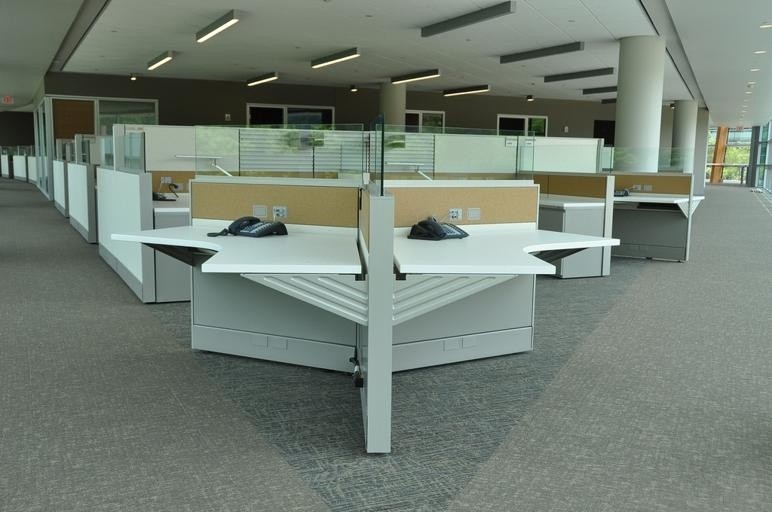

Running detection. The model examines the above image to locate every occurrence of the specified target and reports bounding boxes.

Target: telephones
[228,216,287,237]
[152,192,165,200]
[410,219,469,240]
[614,190,628,196]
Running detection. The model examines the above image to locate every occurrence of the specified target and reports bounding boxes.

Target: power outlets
[633,185,641,190]
[173,183,183,191]
[272,207,287,217]
[449,208,463,220]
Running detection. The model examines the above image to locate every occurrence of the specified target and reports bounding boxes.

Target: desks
[109,220,366,329]
[614,193,689,219]
[392,222,622,329]
[693,196,704,214]
[152,193,191,303]
[539,193,605,278]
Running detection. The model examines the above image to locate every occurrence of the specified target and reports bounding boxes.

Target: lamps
[601,98,616,104]
[312,47,360,69]
[147,51,173,72]
[583,86,617,94]
[544,68,613,82]
[500,42,584,63]
[670,104,674,108]
[422,0,516,38]
[247,72,279,86]
[443,84,489,96]
[391,68,440,84]
[350,86,357,92]
[527,95,534,101]
[196,9,238,43]
